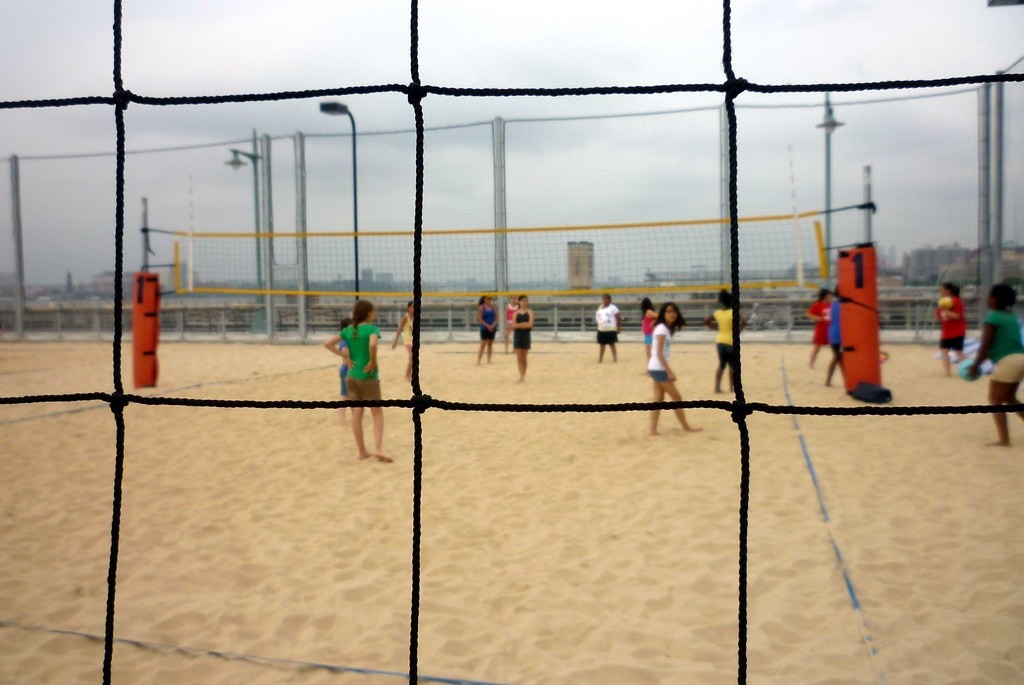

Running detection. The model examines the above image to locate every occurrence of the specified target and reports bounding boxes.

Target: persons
[594,292,621,362]
[338,317,353,425]
[640,296,659,357]
[475,295,499,365]
[823,285,843,388]
[963,283,1024,446]
[806,288,833,368]
[503,294,519,354]
[324,302,394,462]
[935,282,966,379]
[705,287,747,392]
[644,301,703,438]
[512,295,534,385]
[392,301,412,383]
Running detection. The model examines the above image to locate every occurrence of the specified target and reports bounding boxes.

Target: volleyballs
[938,296,952,308]
[958,359,982,382]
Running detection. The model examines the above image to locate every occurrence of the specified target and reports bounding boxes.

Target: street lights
[227,126,264,305]
[319,102,360,302]
[815,91,846,287]
